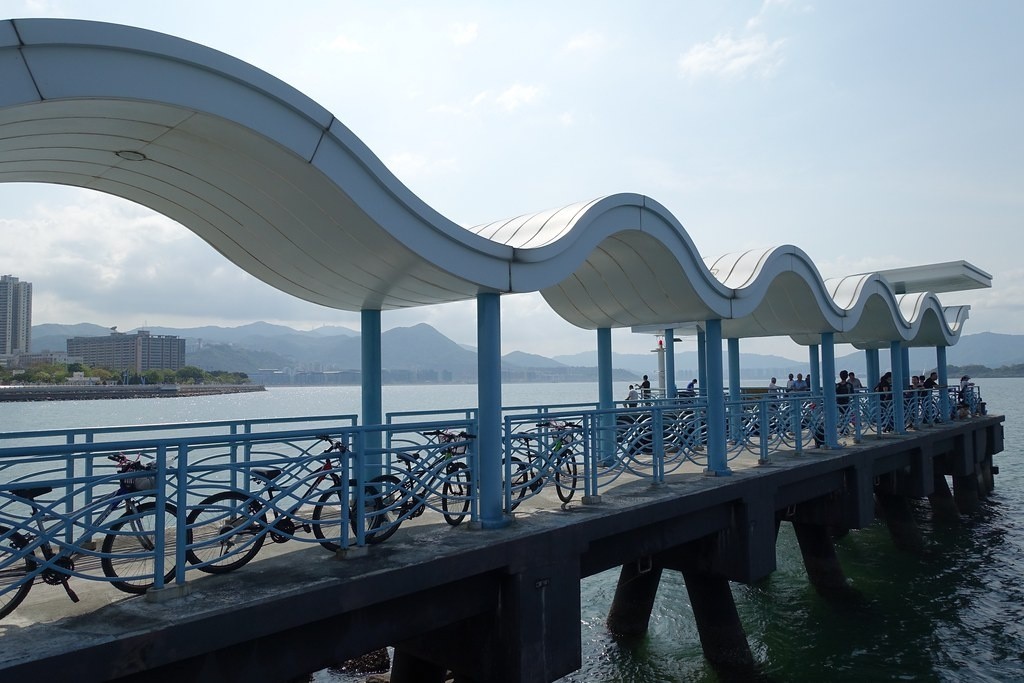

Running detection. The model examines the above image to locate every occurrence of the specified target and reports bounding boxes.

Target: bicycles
[501,422,582,514]
[184,431,384,576]
[350,430,478,546]
[0,452,194,623]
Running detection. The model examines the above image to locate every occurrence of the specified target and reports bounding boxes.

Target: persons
[958,375,974,418]
[874,371,942,432]
[622,375,652,407]
[686,379,698,398]
[834,370,863,435]
[768,369,812,397]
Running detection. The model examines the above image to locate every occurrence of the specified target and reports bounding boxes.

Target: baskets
[439,435,466,454]
[550,428,572,444]
[123,465,156,492]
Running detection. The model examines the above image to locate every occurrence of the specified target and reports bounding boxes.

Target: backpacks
[836,383,848,403]
